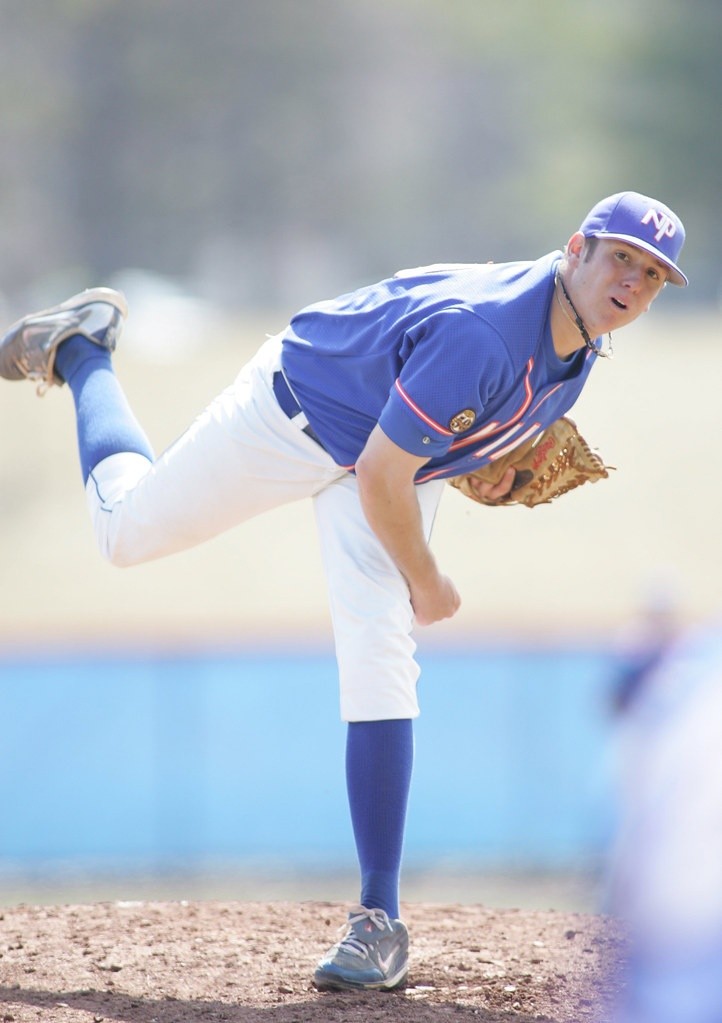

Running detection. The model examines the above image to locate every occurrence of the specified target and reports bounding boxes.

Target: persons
[0,188,687,993]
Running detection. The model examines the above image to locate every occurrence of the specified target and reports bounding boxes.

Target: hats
[578,191,689,288]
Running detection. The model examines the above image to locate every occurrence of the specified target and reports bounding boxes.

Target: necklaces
[553,263,616,362]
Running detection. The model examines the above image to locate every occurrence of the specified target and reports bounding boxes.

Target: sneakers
[308,903,411,993]
[0,286,129,397]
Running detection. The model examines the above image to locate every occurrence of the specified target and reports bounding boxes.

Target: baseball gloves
[446,414,618,509]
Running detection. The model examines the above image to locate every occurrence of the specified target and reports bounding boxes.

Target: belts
[269,370,324,449]
